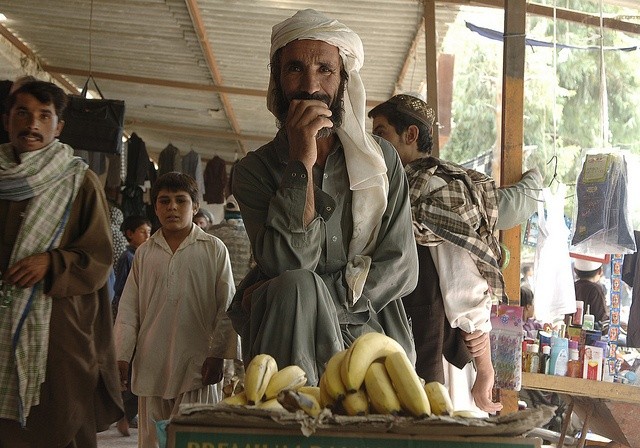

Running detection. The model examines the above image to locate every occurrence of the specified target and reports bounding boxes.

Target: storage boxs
[167,404,542,448]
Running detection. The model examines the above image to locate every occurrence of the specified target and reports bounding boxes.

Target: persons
[210,195,257,290]
[494,168,543,230]
[228,8,418,387]
[1,75,124,447]
[115,210,153,437]
[193,207,214,232]
[116,172,240,447]
[521,264,533,285]
[368,94,505,415]
[572,257,610,335]
[521,287,543,336]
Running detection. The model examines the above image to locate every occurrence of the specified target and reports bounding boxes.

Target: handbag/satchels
[63,94,125,157]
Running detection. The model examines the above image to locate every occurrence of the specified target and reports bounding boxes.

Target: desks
[521,371,640,447]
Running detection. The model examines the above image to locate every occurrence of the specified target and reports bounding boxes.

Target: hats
[387,95,436,131]
[225,195,241,212]
[575,256,602,271]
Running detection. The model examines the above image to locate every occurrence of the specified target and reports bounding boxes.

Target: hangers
[127,131,143,142]
[183,150,200,155]
[160,144,178,150]
[208,156,225,162]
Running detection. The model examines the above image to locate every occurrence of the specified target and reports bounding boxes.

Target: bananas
[222,355,306,416]
[286,334,457,419]
